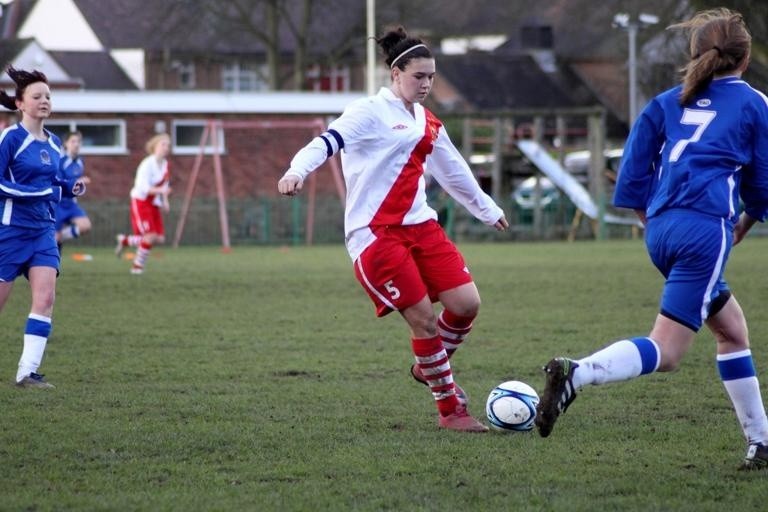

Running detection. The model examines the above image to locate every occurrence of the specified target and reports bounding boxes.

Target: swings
[232,127,301,247]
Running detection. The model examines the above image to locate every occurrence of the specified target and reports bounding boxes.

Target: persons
[1,68,87,391]
[54,131,93,264]
[113,132,174,273]
[278,25,511,434]
[533,12,768,469]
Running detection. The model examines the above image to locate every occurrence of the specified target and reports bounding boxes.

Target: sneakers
[744,443,768,468]
[438,404,490,432]
[16,367,55,389]
[114,233,127,257]
[411,363,468,405]
[130,262,142,274]
[534,355,578,438]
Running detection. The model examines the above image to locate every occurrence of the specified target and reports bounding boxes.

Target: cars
[510,147,628,223]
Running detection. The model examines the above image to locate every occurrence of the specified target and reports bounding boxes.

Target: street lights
[610,10,659,134]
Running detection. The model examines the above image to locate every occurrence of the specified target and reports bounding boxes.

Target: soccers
[486,381,541,433]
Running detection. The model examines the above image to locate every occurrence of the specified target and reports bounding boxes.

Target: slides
[517,137,645,230]
